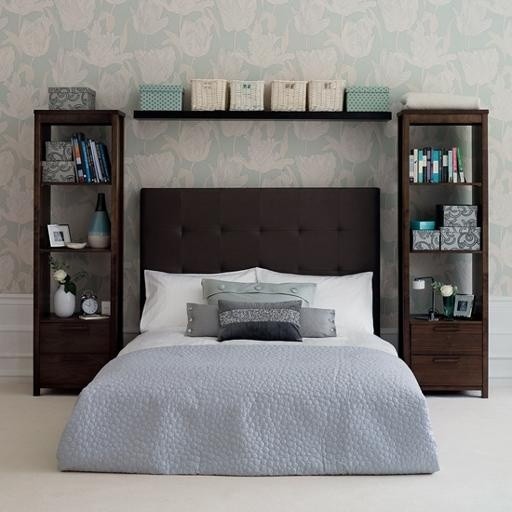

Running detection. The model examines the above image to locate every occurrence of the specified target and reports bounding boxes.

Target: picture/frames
[46,223,71,248]
[453,294,475,318]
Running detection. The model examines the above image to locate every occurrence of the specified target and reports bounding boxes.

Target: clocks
[78,290,99,317]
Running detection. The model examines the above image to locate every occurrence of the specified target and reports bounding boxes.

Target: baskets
[270,80,309,112]
[190,78,229,111]
[229,79,267,111]
[308,78,347,112]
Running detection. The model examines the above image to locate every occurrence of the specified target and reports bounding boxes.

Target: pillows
[139,266,258,334]
[200,278,316,307]
[183,301,336,339]
[216,299,303,342]
[256,265,375,336]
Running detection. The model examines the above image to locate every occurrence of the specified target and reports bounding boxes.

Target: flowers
[48,255,88,295]
[427,278,458,298]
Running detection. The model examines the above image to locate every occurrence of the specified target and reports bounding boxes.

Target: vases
[443,296,454,318]
[87,192,111,249]
[53,285,76,318]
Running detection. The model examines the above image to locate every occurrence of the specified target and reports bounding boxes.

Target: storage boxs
[139,83,185,111]
[344,85,390,112]
[442,204,478,227]
[411,229,440,252]
[439,226,482,252]
[41,160,76,183]
[45,141,73,161]
[48,86,96,111]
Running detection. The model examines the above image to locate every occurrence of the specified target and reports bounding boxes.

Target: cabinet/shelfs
[396,109,490,398]
[32,109,126,396]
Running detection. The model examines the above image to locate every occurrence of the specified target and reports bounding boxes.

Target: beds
[53,187,440,476]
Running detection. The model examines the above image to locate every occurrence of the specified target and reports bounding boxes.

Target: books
[70,132,112,184]
[408,144,466,184]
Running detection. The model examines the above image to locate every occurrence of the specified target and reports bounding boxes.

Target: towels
[399,91,481,110]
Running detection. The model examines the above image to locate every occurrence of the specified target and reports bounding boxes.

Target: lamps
[412,276,440,322]
